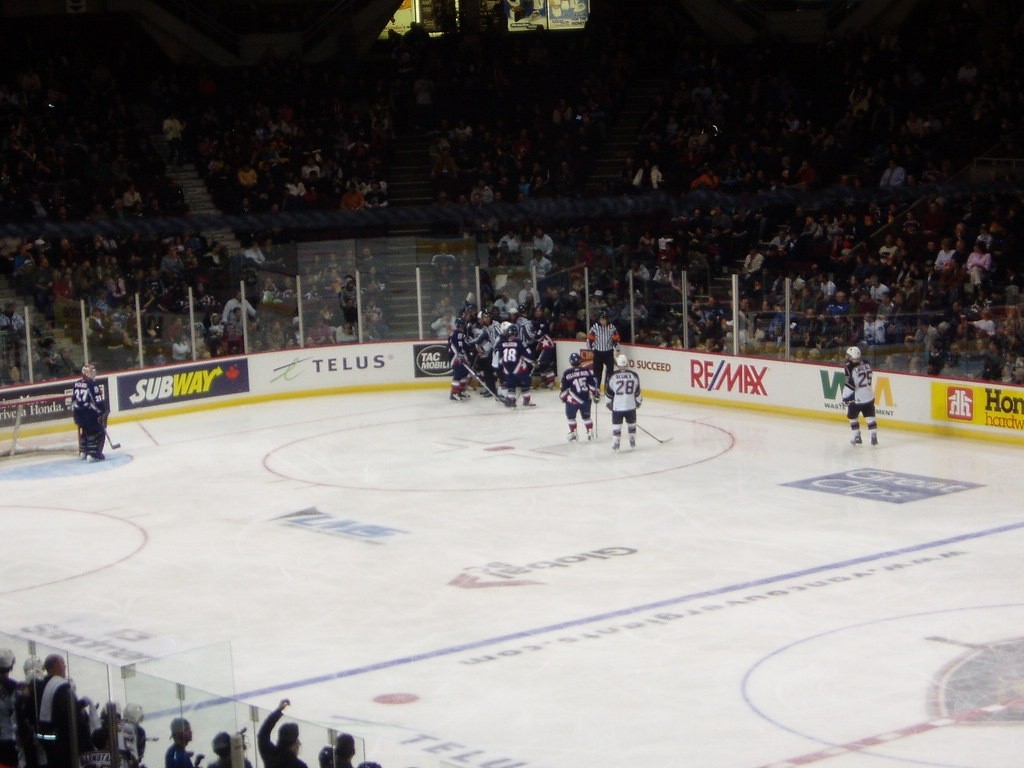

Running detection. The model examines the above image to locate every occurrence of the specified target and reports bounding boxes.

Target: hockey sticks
[517,349,545,398]
[594,377,599,439]
[467,352,481,389]
[636,425,675,445]
[462,362,505,403]
[104,426,121,449]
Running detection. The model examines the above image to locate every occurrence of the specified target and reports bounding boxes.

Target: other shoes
[450,392,470,400]
[850,436,862,447]
[871,434,880,449]
[510,399,517,410]
[480,390,497,397]
[523,393,536,408]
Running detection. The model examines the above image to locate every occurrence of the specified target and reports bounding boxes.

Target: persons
[605,355,643,449]
[447,303,556,408]
[0,0,1024,388]
[587,312,621,391]
[0,647,356,768]
[559,353,601,440]
[73,363,106,462]
[843,346,879,446]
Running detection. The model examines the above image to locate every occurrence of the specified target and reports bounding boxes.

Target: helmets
[82,364,95,382]
[509,308,518,313]
[123,703,143,723]
[847,347,861,359]
[501,321,511,331]
[569,353,579,364]
[617,355,627,366]
[455,318,464,324]
[507,325,517,335]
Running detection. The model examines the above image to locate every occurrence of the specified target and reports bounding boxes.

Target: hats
[0,648,14,667]
[594,290,603,296]
[569,291,577,297]
